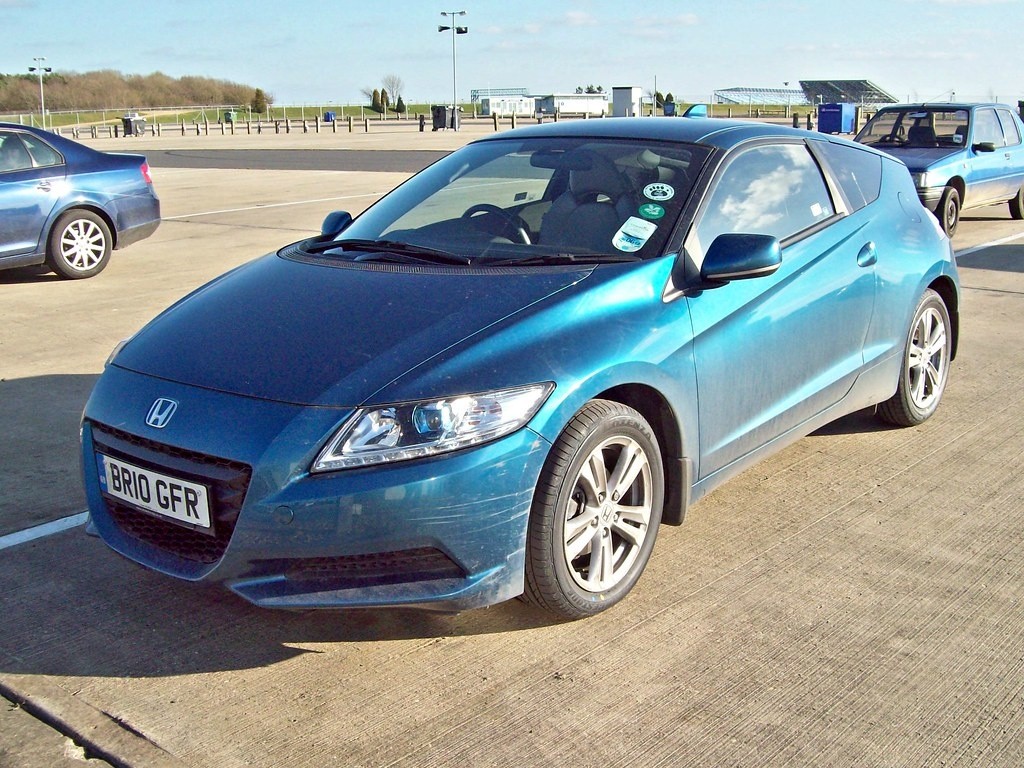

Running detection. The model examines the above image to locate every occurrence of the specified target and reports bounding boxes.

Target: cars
[0,122,161,280]
[852,103,1024,238]
[78,103,959,624]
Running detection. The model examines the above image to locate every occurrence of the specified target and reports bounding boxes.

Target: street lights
[28,57,51,131]
[437,10,469,132]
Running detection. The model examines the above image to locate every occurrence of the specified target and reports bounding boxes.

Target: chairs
[952,125,967,145]
[541,154,636,255]
[906,126,936,146]
